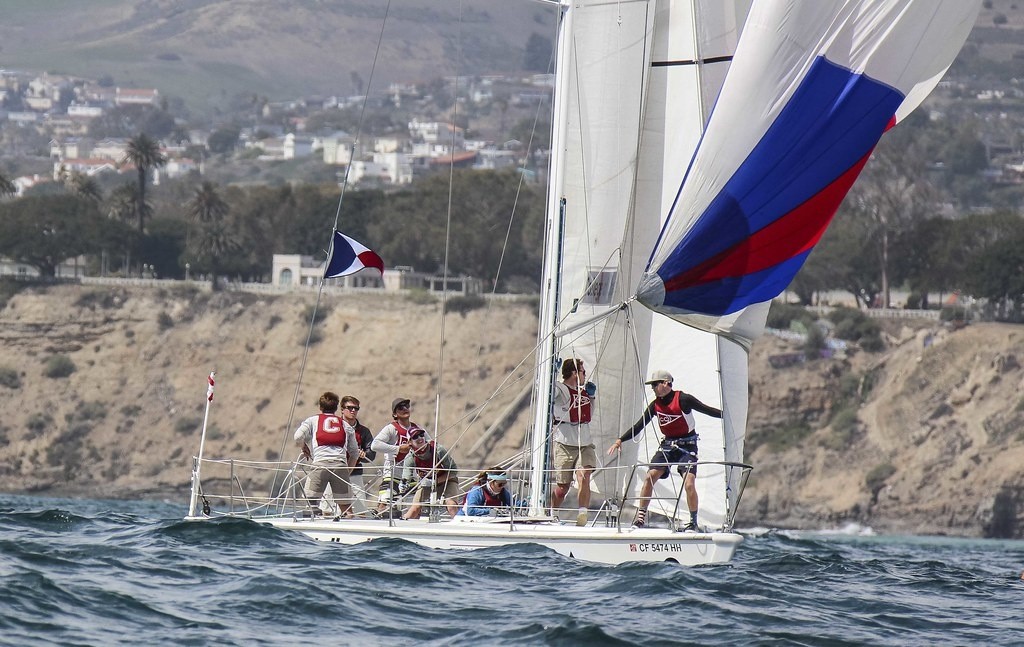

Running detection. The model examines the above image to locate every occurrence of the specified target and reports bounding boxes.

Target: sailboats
[178,0,974,568]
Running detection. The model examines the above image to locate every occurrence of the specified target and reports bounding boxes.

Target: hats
[406,427,425,441]
[645,370,673,385]
[392,398,410,413]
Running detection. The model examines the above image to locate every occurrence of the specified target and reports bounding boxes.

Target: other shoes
[575,513,587,526]
[685,525,699,532]
[631,521,644,528]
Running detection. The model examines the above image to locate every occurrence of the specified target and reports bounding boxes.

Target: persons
[607,369,723,533]
[399,427,458,520]
[318,396,376,517]
[294,392,359,517]
[371,397,432,514]
[456,467,530,516]
[551,359,596,526]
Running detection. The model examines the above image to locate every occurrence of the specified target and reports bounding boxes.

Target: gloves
[398,481,410,493]
[585,381,596,395]
[420,478,432,486]
[556,358,562,369]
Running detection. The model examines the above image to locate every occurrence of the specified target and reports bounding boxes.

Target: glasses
[496,481,506,487]
[396,403,409,410]
[345,406,360,411]
[651,382,663,386]
[580,370,585,375]
[412,432,424,440]
